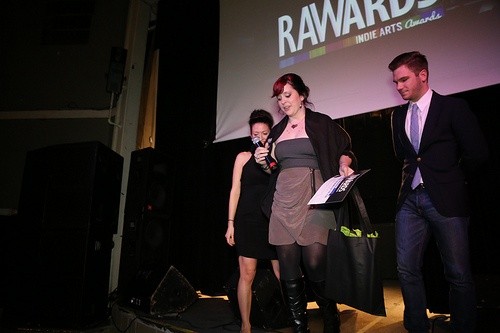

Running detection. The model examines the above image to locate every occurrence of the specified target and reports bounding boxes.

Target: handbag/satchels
[324,183,388,317]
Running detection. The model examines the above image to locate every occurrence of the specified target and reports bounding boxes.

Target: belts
[416,183,426,189]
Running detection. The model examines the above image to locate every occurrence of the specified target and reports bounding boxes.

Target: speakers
[106,47,128,94]
[118,264,204,319]
[225,278,294,330]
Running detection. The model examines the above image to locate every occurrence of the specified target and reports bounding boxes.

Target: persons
[224,110,280,333]
[254,73,357,333]
[373,52,480,333]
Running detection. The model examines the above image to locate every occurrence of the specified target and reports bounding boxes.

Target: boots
[280,275,311,333]
[308,277,341,333]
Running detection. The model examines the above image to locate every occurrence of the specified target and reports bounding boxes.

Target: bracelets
[263,166,270,170]
[228,220,234,221]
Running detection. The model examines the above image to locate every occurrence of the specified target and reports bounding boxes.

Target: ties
[410,103,421,189]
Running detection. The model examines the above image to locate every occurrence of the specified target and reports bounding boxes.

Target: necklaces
[288,119,305,128]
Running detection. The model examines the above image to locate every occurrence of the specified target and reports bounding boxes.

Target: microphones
[251,137,278,170]
